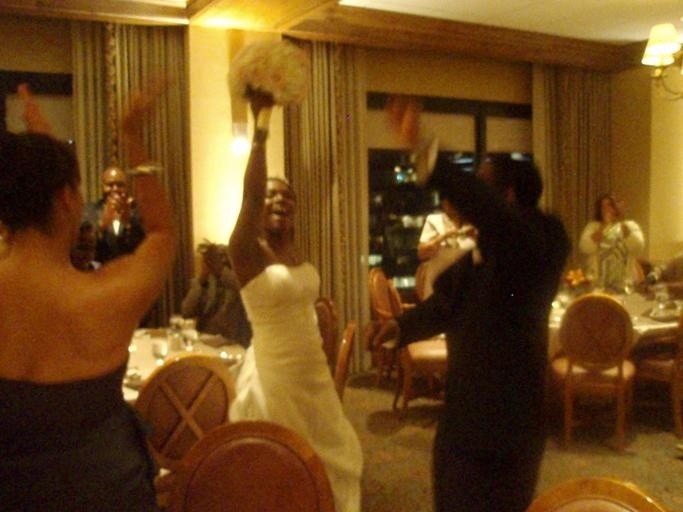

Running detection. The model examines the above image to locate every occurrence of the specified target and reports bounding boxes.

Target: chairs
[354,265,419,380]
[515,475,660,512]
[555,291,638,447]
[130,349,236,508]
[321,319,372,402]
[381,278,455,420]
[167,416,329,510]
[313,295,341,365]
[641,298,680,433]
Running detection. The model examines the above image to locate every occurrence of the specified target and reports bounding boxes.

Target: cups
[150,317,196,365]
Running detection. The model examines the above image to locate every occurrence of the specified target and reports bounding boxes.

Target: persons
[370,88,576,511]
[224,80,365,512]
[572,190,645,286]
[69,221,102,272]
[1,69,193,507]
[179,236,243,342]
[75,158,146,269]
[413,192,482,264]
[474,150,511,206]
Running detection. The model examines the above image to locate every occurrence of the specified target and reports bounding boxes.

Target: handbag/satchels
[415,245,475,304]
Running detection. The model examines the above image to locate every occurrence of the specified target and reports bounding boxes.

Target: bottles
[641,264,667,286]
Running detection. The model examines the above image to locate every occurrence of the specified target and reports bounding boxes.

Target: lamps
[637,11,681,104]
